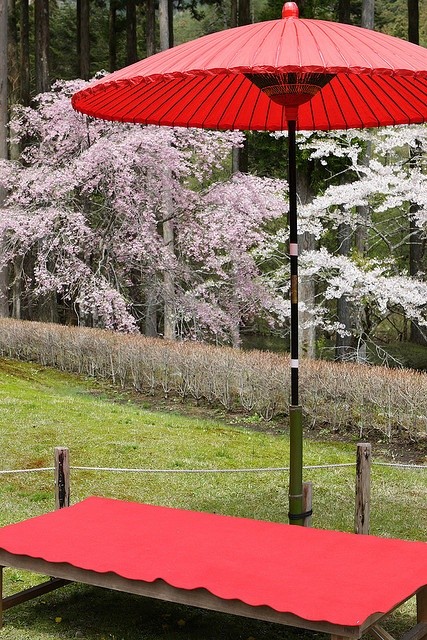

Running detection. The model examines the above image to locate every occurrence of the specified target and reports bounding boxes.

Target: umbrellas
[71,0,427,528]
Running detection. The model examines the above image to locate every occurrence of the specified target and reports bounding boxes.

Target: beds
[0,496,427,639]
